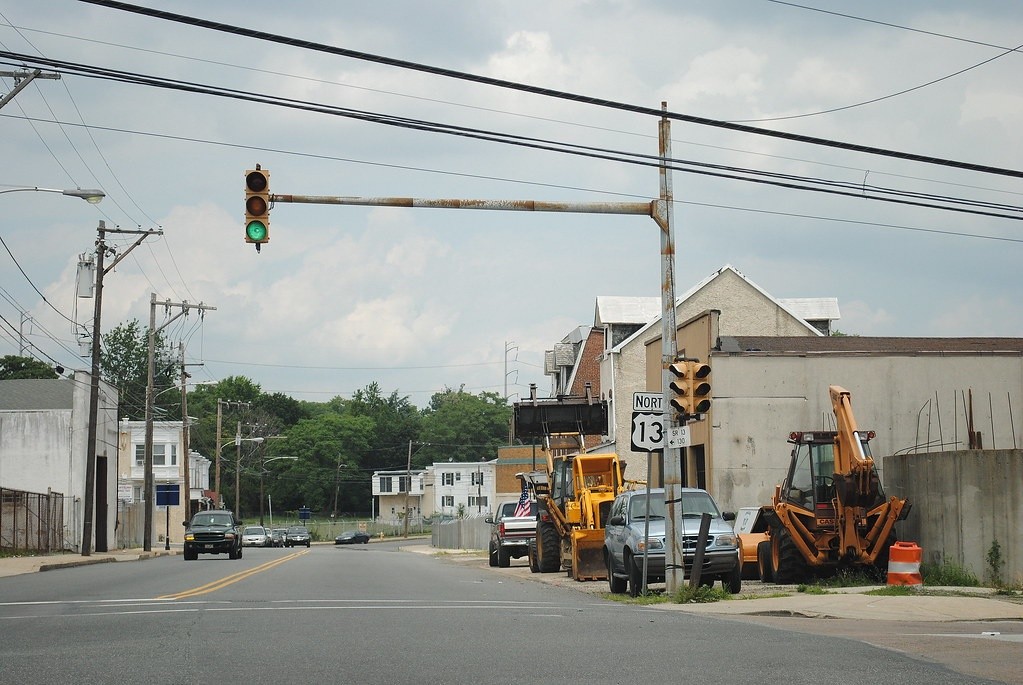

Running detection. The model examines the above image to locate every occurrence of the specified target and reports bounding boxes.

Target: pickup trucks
[486,500,538,566]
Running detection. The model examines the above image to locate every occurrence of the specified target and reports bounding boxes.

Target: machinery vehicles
[512,383,625,581]
[738,385,912,584]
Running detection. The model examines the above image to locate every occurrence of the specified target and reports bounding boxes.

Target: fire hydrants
[378,531,384,540]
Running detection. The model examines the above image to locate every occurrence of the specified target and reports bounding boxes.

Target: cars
[334,530,370,544]
[265,528,287,546]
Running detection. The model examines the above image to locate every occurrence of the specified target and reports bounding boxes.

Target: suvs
[604,487,741,596]
[183,511,243,560]
[286,526,310,548]
[242,526,271,547]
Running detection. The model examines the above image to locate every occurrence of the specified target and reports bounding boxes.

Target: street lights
[260,456,298,526]
[143,380,218,553]
[215,436,264,513]
[334,464,348,523]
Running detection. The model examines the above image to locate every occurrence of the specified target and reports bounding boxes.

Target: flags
[512,484,531,517]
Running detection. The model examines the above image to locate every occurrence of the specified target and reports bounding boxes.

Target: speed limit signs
[631,412,663,454]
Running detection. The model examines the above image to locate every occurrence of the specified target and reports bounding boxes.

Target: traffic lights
[245,168,270,242]
[692,362,712,413]
[669,361,692,414]
